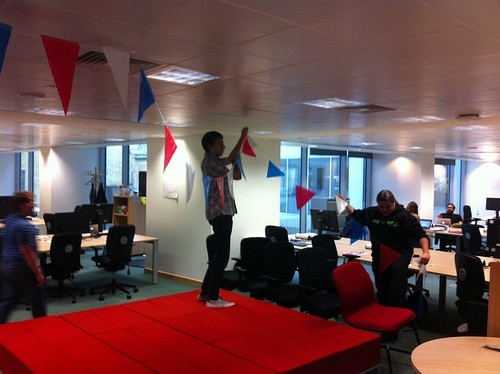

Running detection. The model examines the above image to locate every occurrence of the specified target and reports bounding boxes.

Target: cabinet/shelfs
[112,194,133,226]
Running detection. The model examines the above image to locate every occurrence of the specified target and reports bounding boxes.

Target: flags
[163,125,177,174]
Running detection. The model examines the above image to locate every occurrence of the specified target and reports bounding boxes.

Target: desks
[36,229,159,284]
[288,231,500,315]
[410,336,500,374]
[422,223,488,238]
[25,218,46,226]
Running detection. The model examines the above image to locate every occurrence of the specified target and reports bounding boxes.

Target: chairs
[231,205,500,372]
[43,201,139,304]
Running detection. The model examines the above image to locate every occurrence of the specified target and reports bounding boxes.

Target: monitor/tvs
[309,209,339,235]
[45,202,116,240]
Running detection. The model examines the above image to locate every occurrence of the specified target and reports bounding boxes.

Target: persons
[198,127,248,306]
[337,190,430,343]
[0,191,48,326]
[438,203,463,252]
[406,201,420,222]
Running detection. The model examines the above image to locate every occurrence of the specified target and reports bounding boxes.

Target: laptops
[419,219,432,231]
[486,198,500,218]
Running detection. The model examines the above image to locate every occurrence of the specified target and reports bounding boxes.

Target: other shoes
[380,332,398,342]
[197,294,209,301]
[207,298,235,309]
